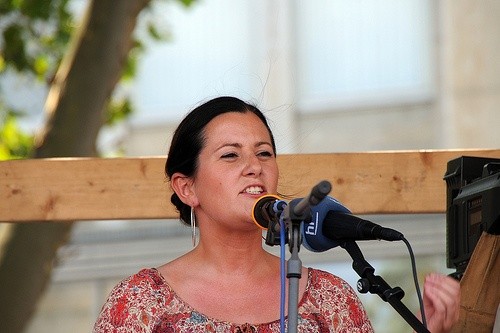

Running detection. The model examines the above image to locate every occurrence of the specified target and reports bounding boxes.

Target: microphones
[300,196,404,253]
[252,195,288,230]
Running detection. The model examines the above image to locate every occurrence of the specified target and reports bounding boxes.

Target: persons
[92,96,460,333]
